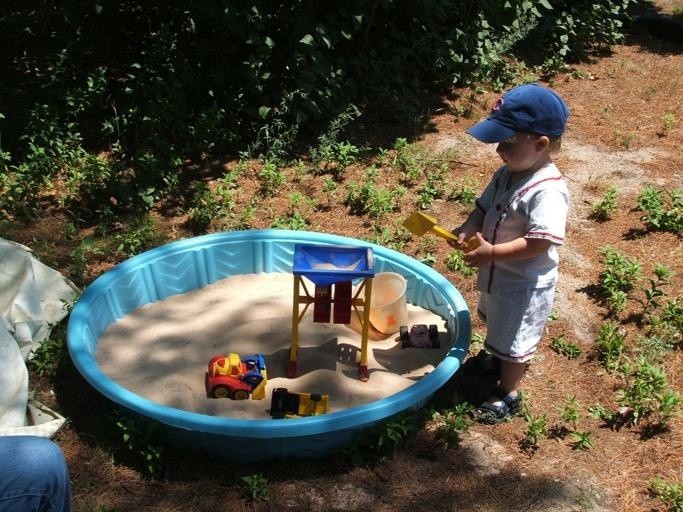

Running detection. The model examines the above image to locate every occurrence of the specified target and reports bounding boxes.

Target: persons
[445,82,571,424]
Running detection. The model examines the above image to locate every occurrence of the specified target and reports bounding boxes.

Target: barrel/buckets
[353,272,408,335]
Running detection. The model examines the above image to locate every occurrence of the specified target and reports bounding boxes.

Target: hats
[463,85,570,147]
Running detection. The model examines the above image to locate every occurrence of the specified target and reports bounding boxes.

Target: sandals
[460,347,499,377]
[476,381,521,421]
